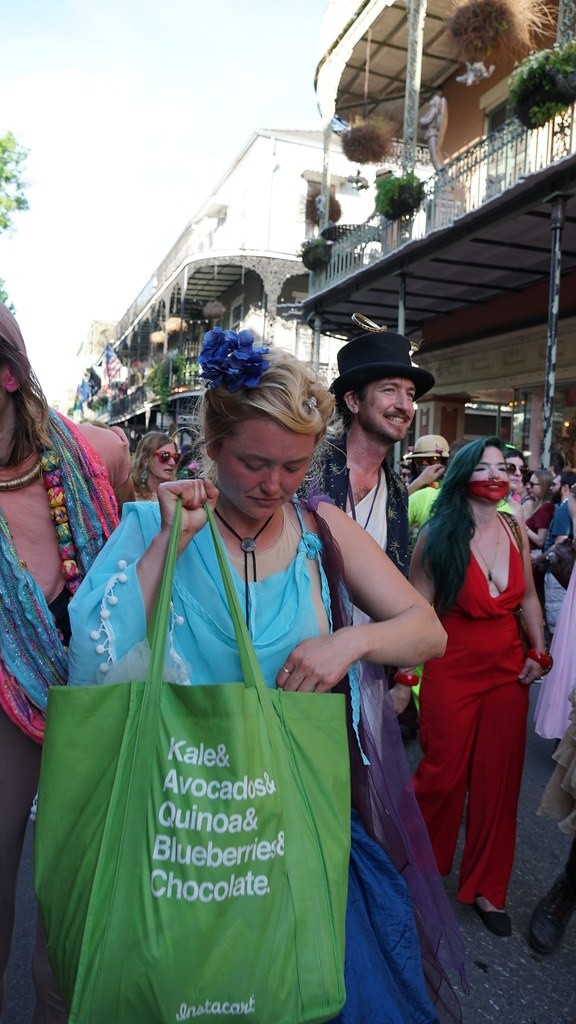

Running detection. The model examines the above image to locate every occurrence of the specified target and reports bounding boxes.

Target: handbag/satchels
[545,516,576,591]
[29,498,350,1024]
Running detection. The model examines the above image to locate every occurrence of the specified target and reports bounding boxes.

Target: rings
[282,666,291,674]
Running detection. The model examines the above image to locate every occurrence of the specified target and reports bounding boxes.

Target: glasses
[414,457,443,466]
[529,482,542,488]
[154,450,183,465]
[506,462,530,475]
[550,482,562,486]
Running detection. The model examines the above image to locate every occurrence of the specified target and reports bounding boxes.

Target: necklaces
[214,508,275,631]
[0,446,82,602]
[464,516,499,581]
[346,466,382,530]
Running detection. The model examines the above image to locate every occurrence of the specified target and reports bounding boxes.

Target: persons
[78,419,182,502]
[0,301,136,1024]
[419,95,445,172]
[292,333,547,935]
[68,329,469,1023]
[525,415,576,957]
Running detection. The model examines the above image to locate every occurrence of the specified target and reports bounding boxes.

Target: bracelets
[527,649,550,671]
[394,672,418,686]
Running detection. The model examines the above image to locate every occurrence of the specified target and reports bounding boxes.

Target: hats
[403,434,452,459]
[328,331,436,413]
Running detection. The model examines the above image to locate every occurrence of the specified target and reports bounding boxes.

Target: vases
[516,68,576,129]
[301,244,331,270]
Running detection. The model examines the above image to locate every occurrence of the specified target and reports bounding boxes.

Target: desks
[321,224,379,255]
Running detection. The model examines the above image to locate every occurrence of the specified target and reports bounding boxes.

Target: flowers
[198,326,271,393]
[507,39,576,109]
[295,238,334,258]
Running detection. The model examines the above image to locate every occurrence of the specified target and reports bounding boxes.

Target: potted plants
[146,356,186,413]
[376,171,423,222]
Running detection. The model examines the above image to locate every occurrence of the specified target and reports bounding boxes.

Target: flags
[74,373,101,412]
[104,338,122,383]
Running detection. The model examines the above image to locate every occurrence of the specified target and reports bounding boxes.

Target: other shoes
[396,715,418,741]
[529,871,576,954]
[471,893,512,938]
